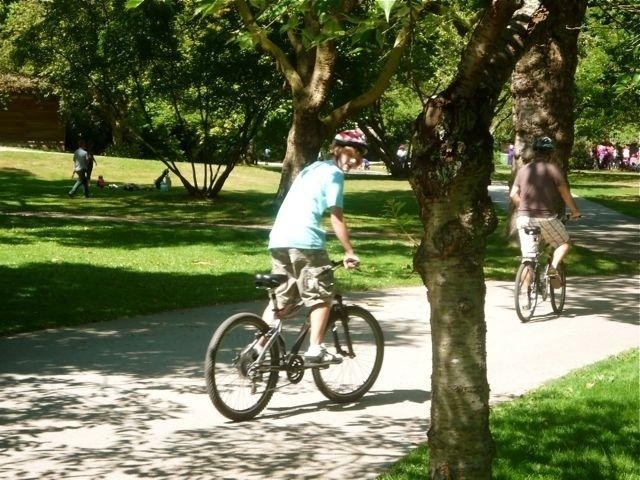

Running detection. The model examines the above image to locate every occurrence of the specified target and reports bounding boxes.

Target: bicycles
[203,259,385,422]
[513,211,584,323]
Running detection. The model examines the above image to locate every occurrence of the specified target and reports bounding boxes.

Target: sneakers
[547,264,562,289]
[304,349,341,367]
[239,350,263,382]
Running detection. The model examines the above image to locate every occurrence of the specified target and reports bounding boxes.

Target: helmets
[334,128,367,154]
[531,135,556,150]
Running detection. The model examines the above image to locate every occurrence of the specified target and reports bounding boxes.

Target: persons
[591,144,640,172]
[237,128,369,380]
[263,147,271,165]
[68,139,90,197]
[96,175,105,188]
[360,158,371,170]
[396,145,407,168]
[86,153,97,179]
[507,144,514,170]
[155,169,169,191]
[509,135,581,311]
